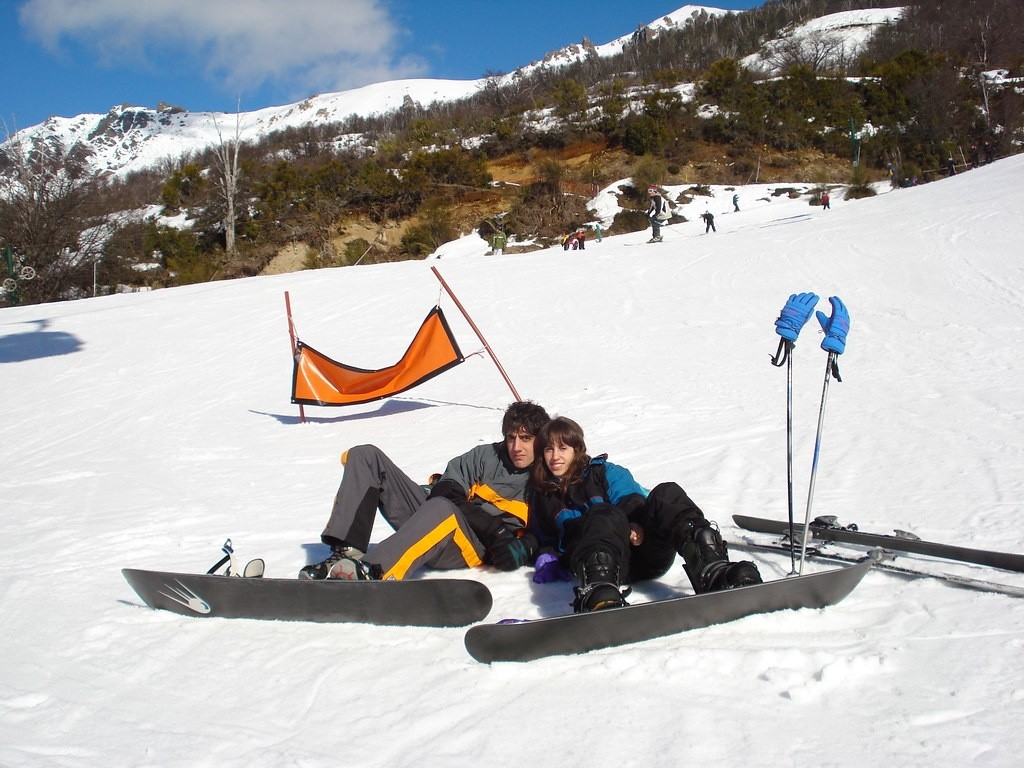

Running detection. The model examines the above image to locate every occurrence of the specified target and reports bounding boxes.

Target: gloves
[773,292,820,341]
[648,216,655,223]
[487,526,537,572]
[816,295,850,354]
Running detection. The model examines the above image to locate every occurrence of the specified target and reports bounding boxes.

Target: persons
[561,224,602,250]
[733,194,740,212]
[645,186,673,243]
[822,193,830,210]
[704,210,716,234]
[527,416,764,613]
[971,141,992,168]
[885,163,931,188]
[298,400,551,583]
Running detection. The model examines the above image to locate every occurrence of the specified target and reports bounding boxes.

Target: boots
[682,522,763,595]
[568,550,628,612]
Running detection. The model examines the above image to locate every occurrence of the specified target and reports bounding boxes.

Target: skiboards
[727,514,1024,599]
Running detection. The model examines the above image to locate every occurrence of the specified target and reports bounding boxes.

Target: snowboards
[465,559,876,664]
[121,567,491,628]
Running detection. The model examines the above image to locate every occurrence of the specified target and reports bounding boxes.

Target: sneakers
[330,557,384,582]
[650,235,660,243]
[299,544,367,580]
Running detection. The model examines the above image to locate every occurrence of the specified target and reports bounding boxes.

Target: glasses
[647,189,653,193]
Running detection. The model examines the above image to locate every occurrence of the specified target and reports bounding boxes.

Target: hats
[647,186,657,191]
